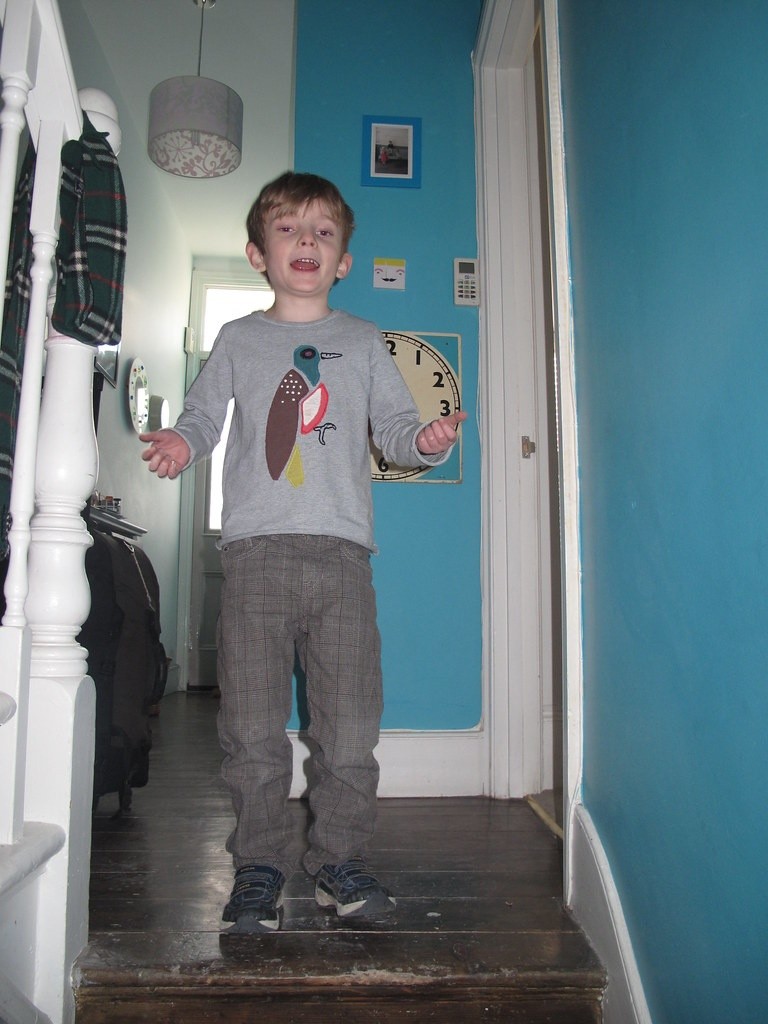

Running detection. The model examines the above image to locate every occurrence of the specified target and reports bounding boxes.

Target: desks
[89,506,149,541]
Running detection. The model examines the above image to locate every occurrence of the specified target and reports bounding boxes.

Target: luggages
[0,373,167,809]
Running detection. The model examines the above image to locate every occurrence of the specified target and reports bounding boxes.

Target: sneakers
[220,865,286,934]
[315,860,396,919]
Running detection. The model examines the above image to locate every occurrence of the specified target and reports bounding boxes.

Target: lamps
[146,0,244,180]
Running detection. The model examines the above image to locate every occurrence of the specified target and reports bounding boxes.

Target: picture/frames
[360,115,423,189]
[93,339,121,390]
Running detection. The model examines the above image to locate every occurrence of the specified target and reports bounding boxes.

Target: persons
[138,172,468,933]
[375,140,394,166]
